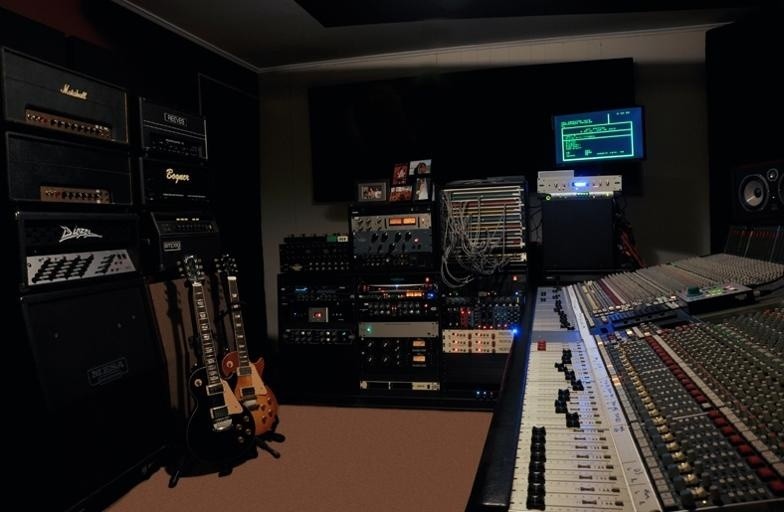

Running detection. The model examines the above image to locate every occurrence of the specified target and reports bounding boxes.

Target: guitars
[216,254,277,440]
[176,255,256,461]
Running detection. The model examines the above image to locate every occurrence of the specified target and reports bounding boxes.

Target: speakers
[728,159,784,223]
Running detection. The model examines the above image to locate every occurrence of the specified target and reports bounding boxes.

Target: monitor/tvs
[551,107,647,168]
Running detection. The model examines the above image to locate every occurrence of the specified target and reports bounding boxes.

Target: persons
[362,161,427,202]
